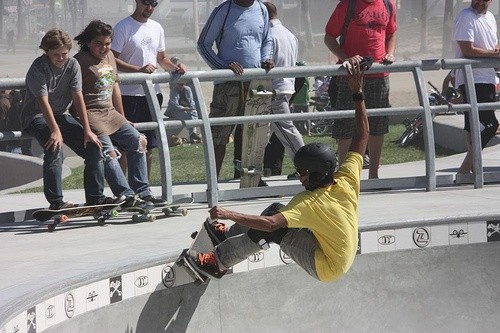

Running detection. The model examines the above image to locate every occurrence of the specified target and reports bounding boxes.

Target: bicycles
[306,95,333,135]
[398,82,464,148]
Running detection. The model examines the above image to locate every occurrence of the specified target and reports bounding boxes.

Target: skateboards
[31,200,126,232]
[122,197,194,223]
[176,217,217,286]
[240,80,280,187]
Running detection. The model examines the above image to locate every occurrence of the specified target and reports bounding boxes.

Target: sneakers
[185,250,227,281]
[287,172,301,179]
[206,218,227,244]
[85,196,126,204]
[49,201,74,209]
[142,196,167,206]
[119,195,153,211]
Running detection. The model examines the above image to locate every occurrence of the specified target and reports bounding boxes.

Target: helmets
[294,142,336,190]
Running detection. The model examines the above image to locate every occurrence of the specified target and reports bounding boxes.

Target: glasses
[142,0,157,7]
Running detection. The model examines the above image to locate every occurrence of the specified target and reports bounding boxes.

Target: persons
[0,90,21,130]
[196,0,274,179]
[233,2,305,181]
[185,59,368,282]
[20,29,126,210]
[8,90,25,130]
[289,61,311,137]
[69,19,169,208]
[453,0,500,151]
[164,81,201,142]
[110,1,186,183]
[324,0,398,190]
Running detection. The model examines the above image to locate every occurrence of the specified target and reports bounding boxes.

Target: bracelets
[351,92,366,102]
